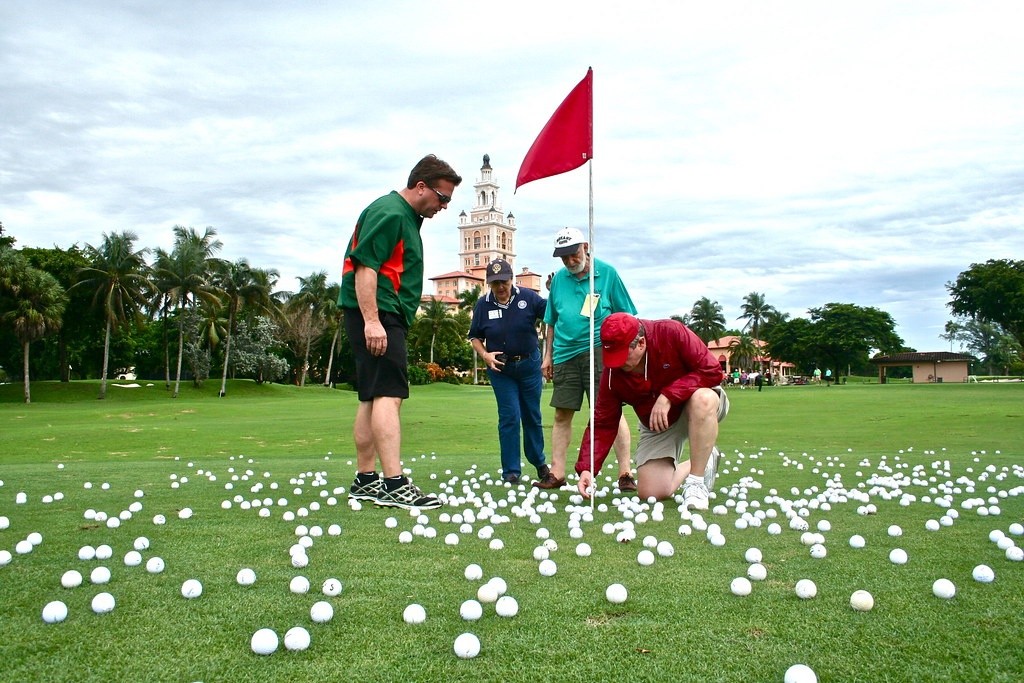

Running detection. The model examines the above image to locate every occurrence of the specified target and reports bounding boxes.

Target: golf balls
[181,579,202,598]
[453,632,481,659]
[488,577,507,595]
[0,438,1024,605]
[61,569,82,588]
[250,627,278,655]
[477,584,498,604]
[90,566,111,584]
[322,578,342,596]
[402,603,426,624]
[460,599,482,620]
[91,592,115,613]
[236,568,256,586]
[41,601,68,623]
[310,601,334,623]
[289,576,310,594]
[283,627,310,653]
[850,589,874,611]
[495,596,518,617]
[783,663,817,683]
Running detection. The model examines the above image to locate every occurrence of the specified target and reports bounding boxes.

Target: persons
[755,372,763,392]
[574,311,731,510]
[469,259,555,483]
[748,369,755,390]
[825,368,832,387]
[740,370,747,390]
[722,370,733,388]
[531,226,637,491]
[733,368,740,389]
[814,366,822,386]
[337,153,461,510]
[764,369,772,386]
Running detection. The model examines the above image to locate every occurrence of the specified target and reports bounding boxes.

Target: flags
[515,69,593,192]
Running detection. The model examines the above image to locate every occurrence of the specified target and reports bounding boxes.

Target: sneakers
[618,472,638,490]
[348,477,384,501]
[703,445,721,492]
[533,472,567,490]
[373,477,443,510]
[681,473,709,510]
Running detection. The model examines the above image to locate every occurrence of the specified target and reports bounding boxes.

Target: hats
[742,370,745,372]
[735,369,738,371]
[486,258,513,283]
[553,227,587,257]
[600,312,639,368]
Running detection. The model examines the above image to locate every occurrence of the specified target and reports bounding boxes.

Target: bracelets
[482,352,486,361]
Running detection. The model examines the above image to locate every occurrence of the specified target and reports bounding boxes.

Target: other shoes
[537,465,550,479]
[504,475,520,483]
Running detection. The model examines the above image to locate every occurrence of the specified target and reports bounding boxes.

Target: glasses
[419,179,451,204]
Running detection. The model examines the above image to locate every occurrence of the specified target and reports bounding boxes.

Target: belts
[507,353,530,362]
